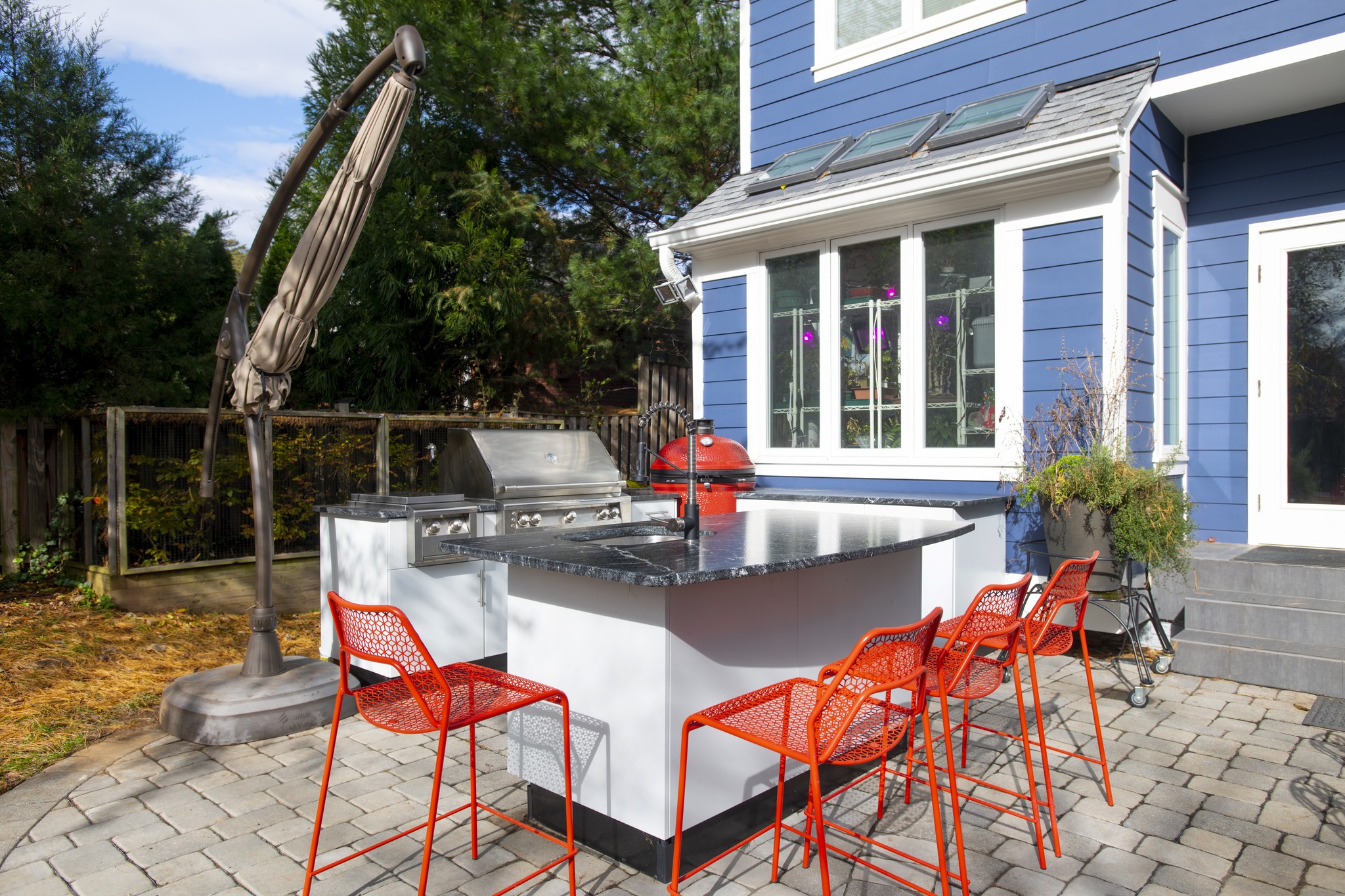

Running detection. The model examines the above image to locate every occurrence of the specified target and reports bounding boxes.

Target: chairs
[804,572,1047,896]
[909,548,1112,857]
[300,591,579,896]
[668,607,954,896]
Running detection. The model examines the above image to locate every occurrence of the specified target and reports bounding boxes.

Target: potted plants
[771,263,995,447]
[996,317,1198,592]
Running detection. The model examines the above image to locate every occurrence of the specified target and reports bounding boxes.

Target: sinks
[554,524,718,548]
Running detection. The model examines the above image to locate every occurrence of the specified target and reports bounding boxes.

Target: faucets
[637,401,703,542]
